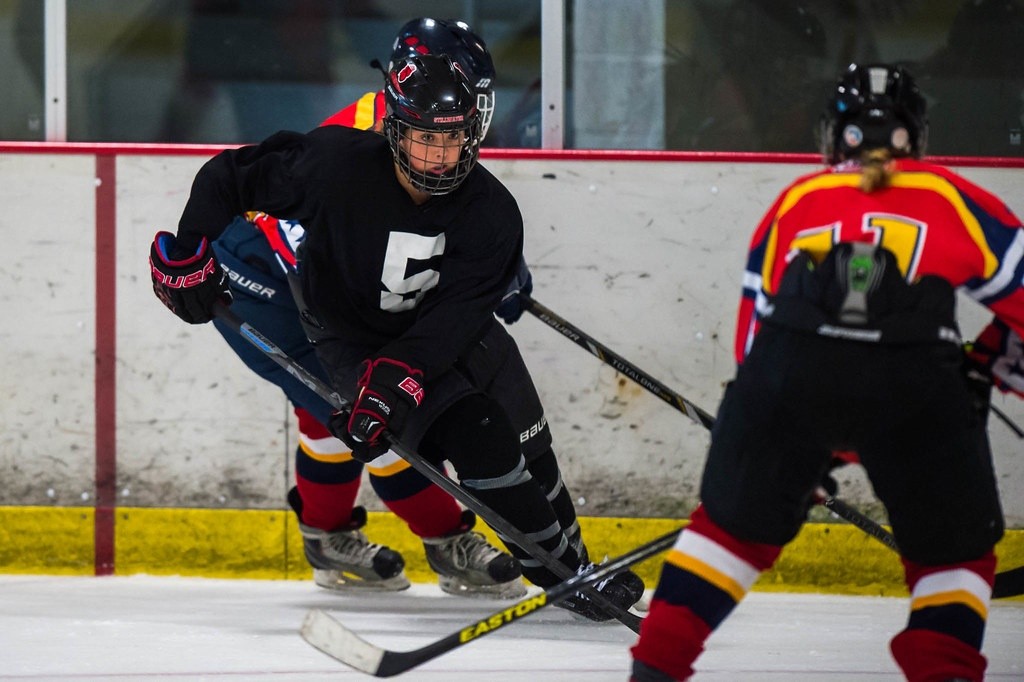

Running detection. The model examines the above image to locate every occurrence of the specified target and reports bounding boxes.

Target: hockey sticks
[296,520,685,677]
[209,302,707,657]
[520,294,1024,603]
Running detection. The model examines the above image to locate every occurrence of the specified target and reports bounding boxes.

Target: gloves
[491,257,532,324]
[327,356,425,462]
[149,229,234,325]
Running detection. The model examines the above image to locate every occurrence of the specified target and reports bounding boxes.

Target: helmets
[388,16,495,157]
[384,42,482,197]
[827,64,929,169]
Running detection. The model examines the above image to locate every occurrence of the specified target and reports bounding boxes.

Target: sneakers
[421,510,529,597]
[286,483,411,591]
[586,561,651,614]
[545,561,633,625]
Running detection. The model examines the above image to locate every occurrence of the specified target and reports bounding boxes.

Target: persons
[629,61,1024,681]
[147,18,647,624]
